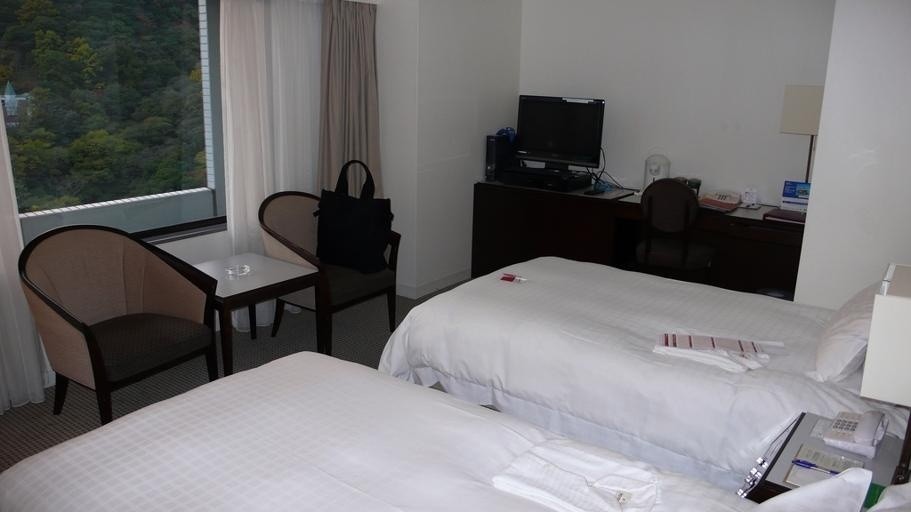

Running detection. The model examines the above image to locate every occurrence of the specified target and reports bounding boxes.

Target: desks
[193,253,320,379]
[613,191,808,302]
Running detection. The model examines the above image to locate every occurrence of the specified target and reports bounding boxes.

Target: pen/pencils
[791,459,839,477]
[746,203,755,207]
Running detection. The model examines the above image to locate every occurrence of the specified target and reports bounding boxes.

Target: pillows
[809,279,882,382]
[749,467,873,510]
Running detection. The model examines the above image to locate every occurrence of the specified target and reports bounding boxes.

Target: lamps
[779,84,825,182]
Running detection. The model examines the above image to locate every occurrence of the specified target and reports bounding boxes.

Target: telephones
[822,411,889,460]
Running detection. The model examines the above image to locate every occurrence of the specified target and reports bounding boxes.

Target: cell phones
[584,190,604,196]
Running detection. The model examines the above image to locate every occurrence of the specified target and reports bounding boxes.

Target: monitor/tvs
[513,95,605,173]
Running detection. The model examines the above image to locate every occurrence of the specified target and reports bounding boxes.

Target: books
[785,443,866,487]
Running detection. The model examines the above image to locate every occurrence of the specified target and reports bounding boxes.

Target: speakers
[642,154,670,192]
[486,135,519,182]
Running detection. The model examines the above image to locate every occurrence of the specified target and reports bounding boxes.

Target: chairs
[20,225,216,424]
[258,190,399,357]
[637,178,715,286]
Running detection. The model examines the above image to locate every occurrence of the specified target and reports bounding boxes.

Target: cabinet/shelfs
[471,179,641,279]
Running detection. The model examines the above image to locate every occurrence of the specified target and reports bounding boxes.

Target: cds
[496,127,515,144]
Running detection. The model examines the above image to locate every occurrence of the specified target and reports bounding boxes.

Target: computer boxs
[505,170,592,190]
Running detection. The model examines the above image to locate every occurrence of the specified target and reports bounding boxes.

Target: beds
[4,350,757,511]
[378,258,907,479]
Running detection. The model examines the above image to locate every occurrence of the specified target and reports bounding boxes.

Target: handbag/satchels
[313,160,394,273]
